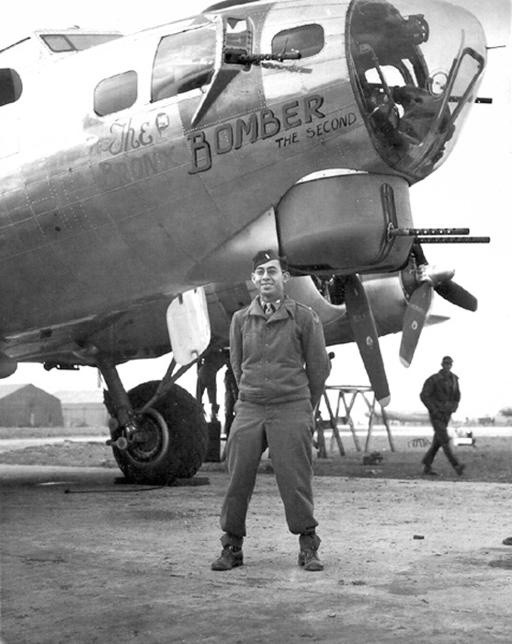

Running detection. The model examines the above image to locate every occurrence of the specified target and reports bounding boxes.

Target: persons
[196,349,227,423]
[419,356,465,475]
[210,249,332,572]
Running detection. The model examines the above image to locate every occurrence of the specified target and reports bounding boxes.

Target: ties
[265,302,273,316]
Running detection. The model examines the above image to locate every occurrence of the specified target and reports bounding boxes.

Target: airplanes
[0,1,495,487]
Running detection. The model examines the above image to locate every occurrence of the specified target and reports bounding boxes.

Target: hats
[252,249,277,270]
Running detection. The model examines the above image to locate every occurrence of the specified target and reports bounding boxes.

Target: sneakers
[299,551,324,570]
[423,465,437,475]
[211,550,243,570]
[457,465,464,475]
